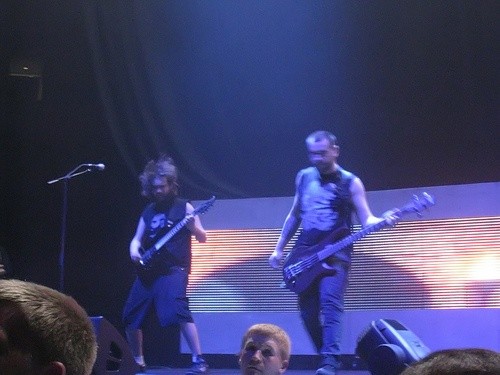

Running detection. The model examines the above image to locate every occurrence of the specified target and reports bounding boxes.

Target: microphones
[83,163,105,171]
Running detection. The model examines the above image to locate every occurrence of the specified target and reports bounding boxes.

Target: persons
[121,156,209,373]
[237,322,292,375]
[268,130,401,375]
[0,279,98,375]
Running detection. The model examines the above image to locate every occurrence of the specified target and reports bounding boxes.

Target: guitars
[280,192,435,295]
[131,196,216,287]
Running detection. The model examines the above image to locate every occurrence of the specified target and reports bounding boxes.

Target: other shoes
[116,358,146,373]
[189,357,208,374]
[315,363,338,375]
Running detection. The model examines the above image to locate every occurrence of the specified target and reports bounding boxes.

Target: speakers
[89,315,139,375]
